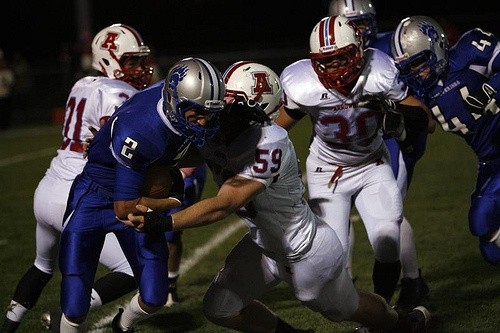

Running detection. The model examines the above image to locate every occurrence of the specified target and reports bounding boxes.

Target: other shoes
[112,308,136,333]
[392,269,430,312]
[406,306,431,332]
[164,275,180,307]
[40,312,61,332]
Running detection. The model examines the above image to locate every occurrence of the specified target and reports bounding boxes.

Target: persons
[0,23,153,333]
[163,0,430,307]
[391,15,500,263]
[60,58,226,333]
[115,60,432,333]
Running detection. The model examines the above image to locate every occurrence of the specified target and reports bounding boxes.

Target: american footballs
[114,164,185,220]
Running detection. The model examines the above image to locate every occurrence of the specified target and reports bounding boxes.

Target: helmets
[223,61,284,123]
[391,16,448,74]
[163,57,225,123]
[91,24,152,80]
[329,0,377,36]
[310,16,364,69]
